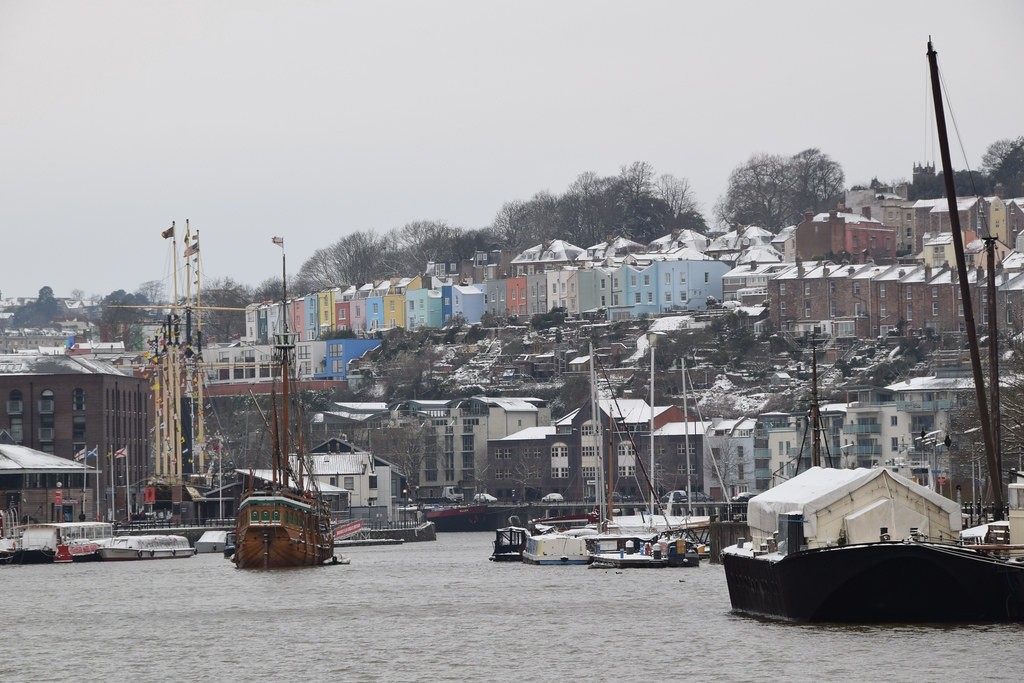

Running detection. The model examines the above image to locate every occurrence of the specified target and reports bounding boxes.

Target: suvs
[473,493,497,504]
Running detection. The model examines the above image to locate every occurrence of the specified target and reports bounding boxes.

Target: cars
[541,493,564,503]
[661,490,688,503]
[582,489,629,505]
[691,492,715,502]
[732,492,757,502]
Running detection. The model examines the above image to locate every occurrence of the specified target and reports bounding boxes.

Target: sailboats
[233,236,334,568]
[488,342,719,569]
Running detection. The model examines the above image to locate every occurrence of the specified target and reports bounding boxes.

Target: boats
[0,512,231,562]
[720,343,1024,626]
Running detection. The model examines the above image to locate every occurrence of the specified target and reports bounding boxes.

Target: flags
[270,236,284,248]
[73,446,126,461]
[214,441,223,452]
[160,224,200,258]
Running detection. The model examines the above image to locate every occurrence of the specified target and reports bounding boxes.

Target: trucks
[418,485,465,506]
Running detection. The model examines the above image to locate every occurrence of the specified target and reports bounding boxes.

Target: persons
[78,511,86,522]
